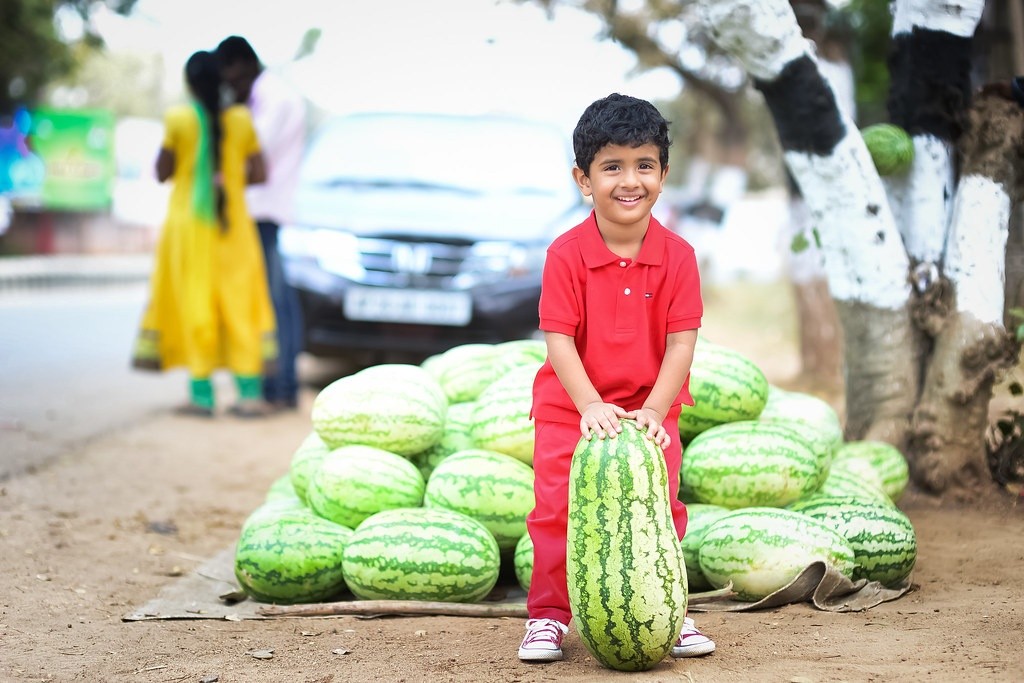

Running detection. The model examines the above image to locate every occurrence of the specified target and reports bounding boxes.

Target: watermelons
[858,123,915,178]
[233,338,919,669]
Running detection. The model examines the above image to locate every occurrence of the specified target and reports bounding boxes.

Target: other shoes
[172,402,213,418]
[228,401,269,417]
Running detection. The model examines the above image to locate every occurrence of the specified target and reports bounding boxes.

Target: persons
[514,89,718,663]
[211,33,307,413]
[125,51,282,419]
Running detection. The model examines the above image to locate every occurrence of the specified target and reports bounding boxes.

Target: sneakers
[518,617,569,659]
[669,617,715,657]
